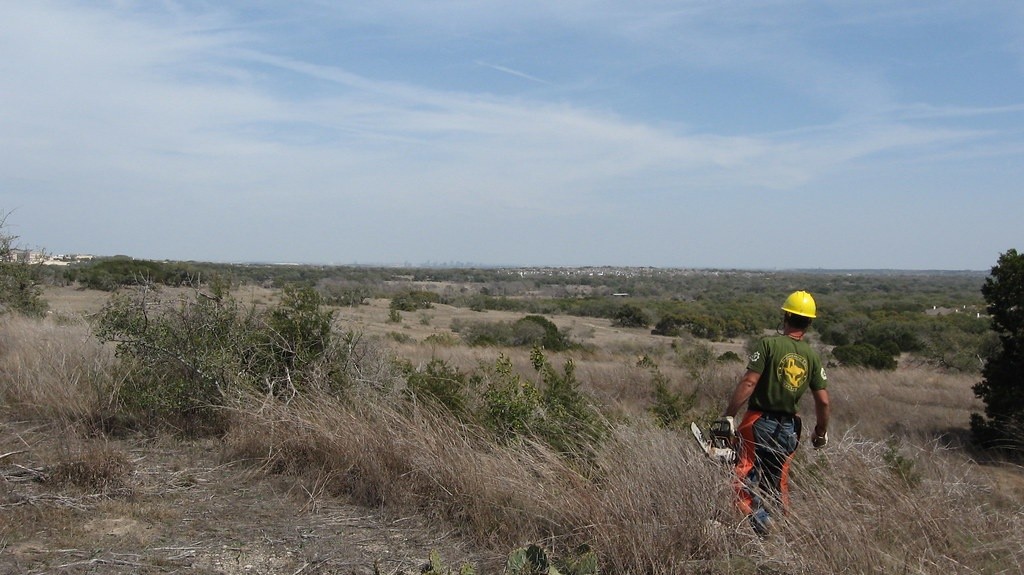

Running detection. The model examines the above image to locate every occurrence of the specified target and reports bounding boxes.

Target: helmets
[780,290,816,318]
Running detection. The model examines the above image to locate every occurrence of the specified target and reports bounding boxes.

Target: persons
[720,290,830,538]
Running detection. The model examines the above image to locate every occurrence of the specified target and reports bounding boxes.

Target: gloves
[811,425,828,451]
[719,418,736,436]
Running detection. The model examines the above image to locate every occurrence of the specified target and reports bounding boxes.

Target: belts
[748,408,795,423]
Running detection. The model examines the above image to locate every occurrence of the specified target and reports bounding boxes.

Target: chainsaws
[689,417,742,464]
